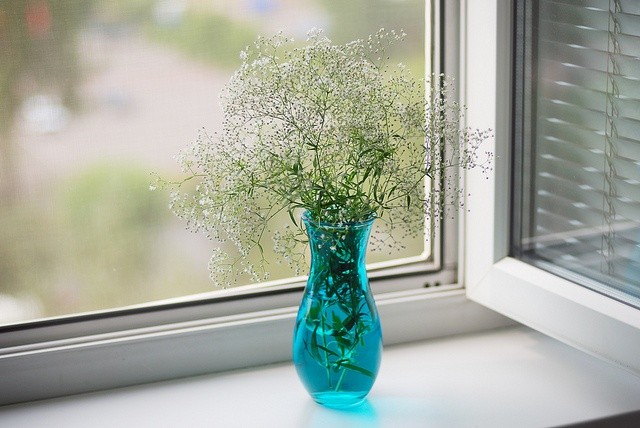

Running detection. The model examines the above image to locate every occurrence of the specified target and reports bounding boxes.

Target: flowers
[147,24,496,392]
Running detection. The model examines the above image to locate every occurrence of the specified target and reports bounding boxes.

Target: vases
[291,211,383,409]
